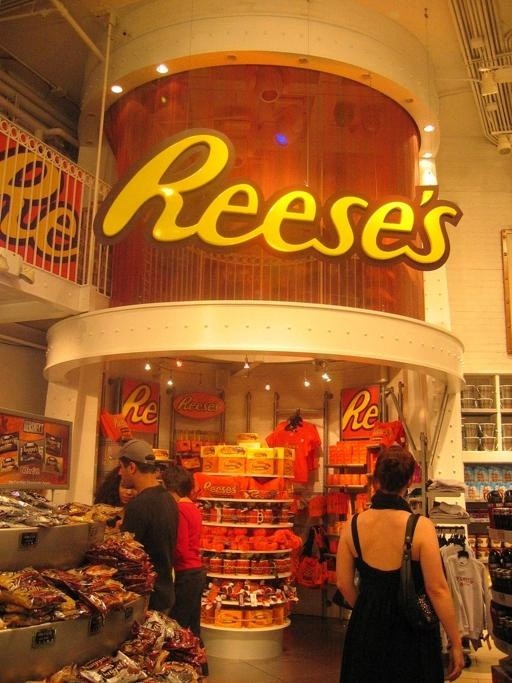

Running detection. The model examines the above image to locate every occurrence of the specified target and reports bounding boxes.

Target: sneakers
[484,489,512,629]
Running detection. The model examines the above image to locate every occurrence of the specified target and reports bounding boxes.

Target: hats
[107,439,157,465]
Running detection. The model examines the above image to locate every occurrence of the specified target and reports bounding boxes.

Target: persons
[162,464,211,683]
[117,438,180,617]
[91,464,141,530]
[336,446,465,682]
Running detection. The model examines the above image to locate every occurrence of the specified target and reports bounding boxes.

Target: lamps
[478,67,499,98]
[498,134,511,157]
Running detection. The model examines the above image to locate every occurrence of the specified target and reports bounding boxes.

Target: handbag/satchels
[398,592,442,637]
